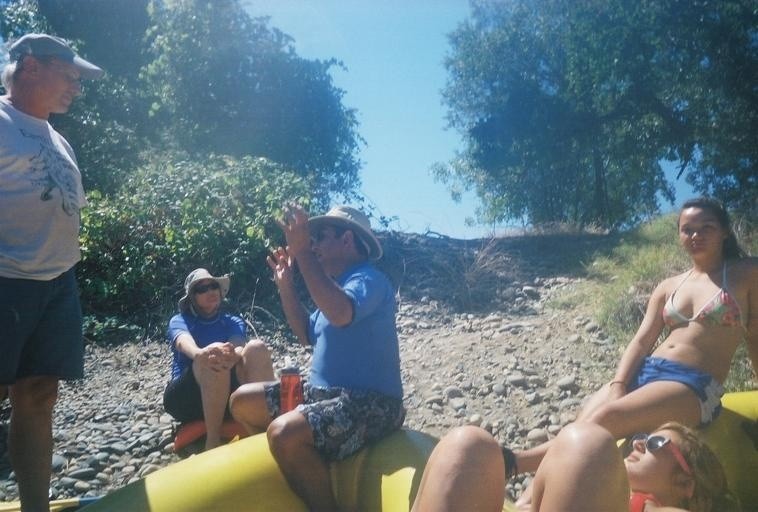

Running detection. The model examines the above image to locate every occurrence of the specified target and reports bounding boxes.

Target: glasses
[193,281,223,293]
[629,433,690,474]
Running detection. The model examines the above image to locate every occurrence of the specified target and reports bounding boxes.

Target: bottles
[280,367,304,415]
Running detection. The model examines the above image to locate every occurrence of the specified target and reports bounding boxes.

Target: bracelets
[610,381,629,388]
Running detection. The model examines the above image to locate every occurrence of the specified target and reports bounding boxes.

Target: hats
[9,34,104,80]
[308,205,383,260]
[179,268,230,312]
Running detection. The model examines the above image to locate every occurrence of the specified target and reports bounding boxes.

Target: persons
[163,268,276,450]
[0,35,102,512]
[481,198,758,512]
[229,202,405,512]
[411,422,741,512]
